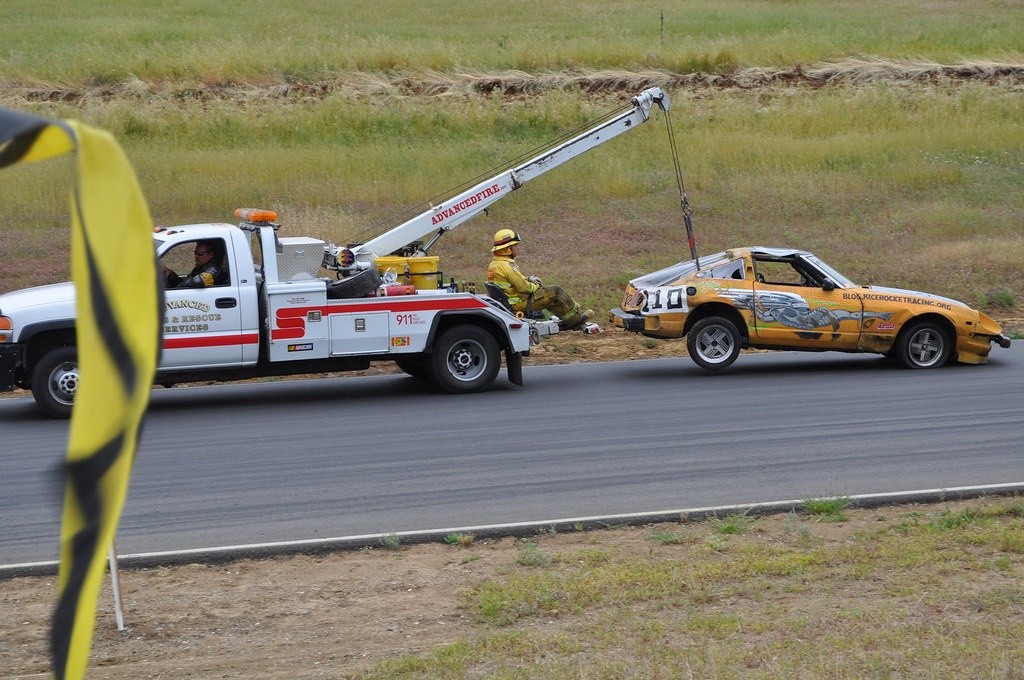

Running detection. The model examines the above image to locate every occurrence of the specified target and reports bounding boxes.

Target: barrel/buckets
[373,254,407,287]
[407,256,439,290]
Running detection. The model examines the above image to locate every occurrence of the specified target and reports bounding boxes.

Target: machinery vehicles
[0,87,673,420]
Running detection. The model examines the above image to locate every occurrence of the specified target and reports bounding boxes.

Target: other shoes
[571,309,595,331]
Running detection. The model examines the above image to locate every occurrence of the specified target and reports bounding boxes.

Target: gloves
[527,275,545,289]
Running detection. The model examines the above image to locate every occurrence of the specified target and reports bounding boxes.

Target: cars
[609,245,1011,371]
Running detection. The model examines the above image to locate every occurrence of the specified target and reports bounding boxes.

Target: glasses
[194,251,208,256]
[514,232,521,241]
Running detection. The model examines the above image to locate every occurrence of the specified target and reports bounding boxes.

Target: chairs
[484,280,548,321]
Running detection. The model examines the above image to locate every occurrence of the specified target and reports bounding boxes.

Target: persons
[487,229,595,331]
[162,243,224,287]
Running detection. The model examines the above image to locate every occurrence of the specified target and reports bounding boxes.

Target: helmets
[491,229,521,252]
